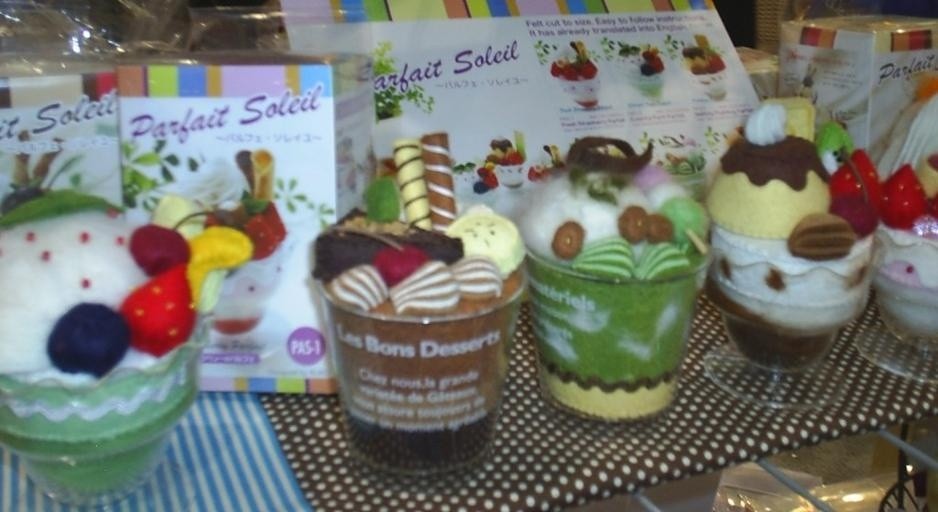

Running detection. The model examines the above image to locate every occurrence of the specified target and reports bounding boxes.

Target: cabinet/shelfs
[0,0,938,512]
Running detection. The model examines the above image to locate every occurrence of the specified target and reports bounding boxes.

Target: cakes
[655,147,708,202]
[2,176,255,509]
[144,147,298,336]
[702,96,888,413]
[488,138,526,189]
[548,40,602,108]
[311,132,530,479]
[678,34,728,104]
[619,45,666,101]
[519,135,716,425]
[855,152,938,387]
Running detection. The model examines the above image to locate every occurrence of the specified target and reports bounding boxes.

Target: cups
[529,247,710,423]
[860,230,938,384]
[0,322,208,502]
[703,244,881,404]
[311,268,527,479]
